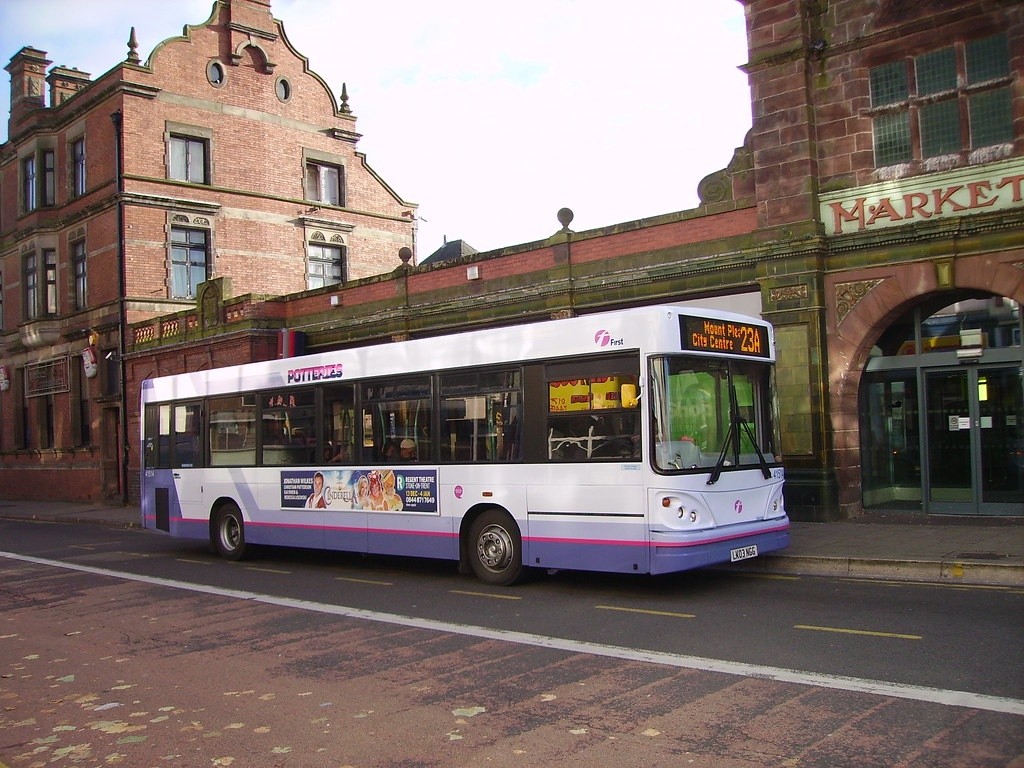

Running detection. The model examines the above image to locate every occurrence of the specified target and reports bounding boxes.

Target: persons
[304,473,328,510]
[304,414,650,465]
[356,471,404,513]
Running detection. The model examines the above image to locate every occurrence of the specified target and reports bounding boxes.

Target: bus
[139,305,790,591]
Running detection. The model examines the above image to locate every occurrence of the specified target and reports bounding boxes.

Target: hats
[400,439,416,448]
